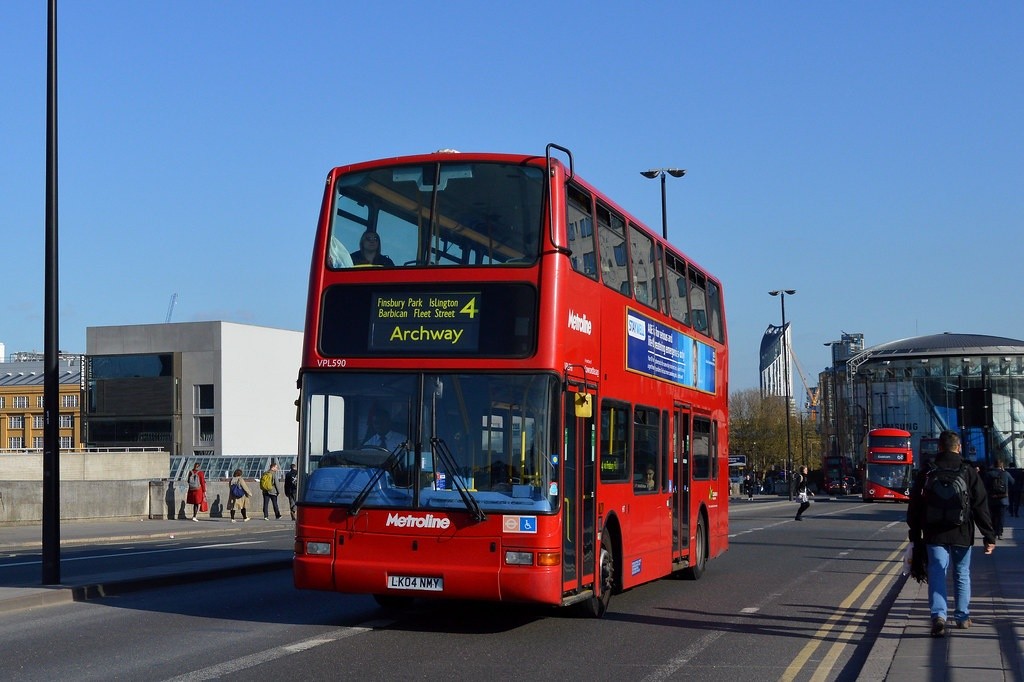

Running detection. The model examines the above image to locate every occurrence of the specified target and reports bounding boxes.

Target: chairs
[404,260,435,265]
[505,258,528,263]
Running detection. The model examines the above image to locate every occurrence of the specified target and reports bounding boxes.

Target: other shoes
[243,517,250,522]
[231,519,236,523]
[264,517,270,521]
[956,619,973,629]
[931,617,946,638]
[192,517,198,522]
[276,515,283,520]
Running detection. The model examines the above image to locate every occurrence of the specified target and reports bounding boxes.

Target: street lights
[768,290,796,501]
[875,392,887,428]
[641,168,686,240]
[824,342,845,489]
[801,412,820,465]
[888,407,900,428]
[859,373,875,432]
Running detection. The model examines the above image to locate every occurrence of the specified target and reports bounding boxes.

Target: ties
[379,436,387,451]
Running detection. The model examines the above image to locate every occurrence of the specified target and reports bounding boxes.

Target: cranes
[785,343,820,426]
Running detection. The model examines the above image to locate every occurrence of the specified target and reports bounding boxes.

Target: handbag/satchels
[230,478,246,499]
[199,496,208,512]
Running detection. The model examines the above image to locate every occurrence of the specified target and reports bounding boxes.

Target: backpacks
[189,470,201,490]
[790,473,803,492]
[285,473,297,489]
[260,471,274,491]
[921,461,970,531]
[985,469,1007,498]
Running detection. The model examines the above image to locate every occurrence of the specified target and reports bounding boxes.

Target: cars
[823,476,862,495]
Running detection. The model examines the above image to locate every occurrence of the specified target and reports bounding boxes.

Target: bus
[920,437,939,472]
[859,428,914,503]
[293,145,730,618]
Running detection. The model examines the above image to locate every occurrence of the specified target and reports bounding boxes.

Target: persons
[1007,463,1021,517]
[285,464,298,520]
[907,431,996,636]
[793,466,814,521]
[744,475,754,501]
[361,409,414,471]
[638,466,655,491]
[186,463,206,522]
[350,230,395,266]
[263,463,283,521]
[227,469,252,522]
[984,458,1015,540]
